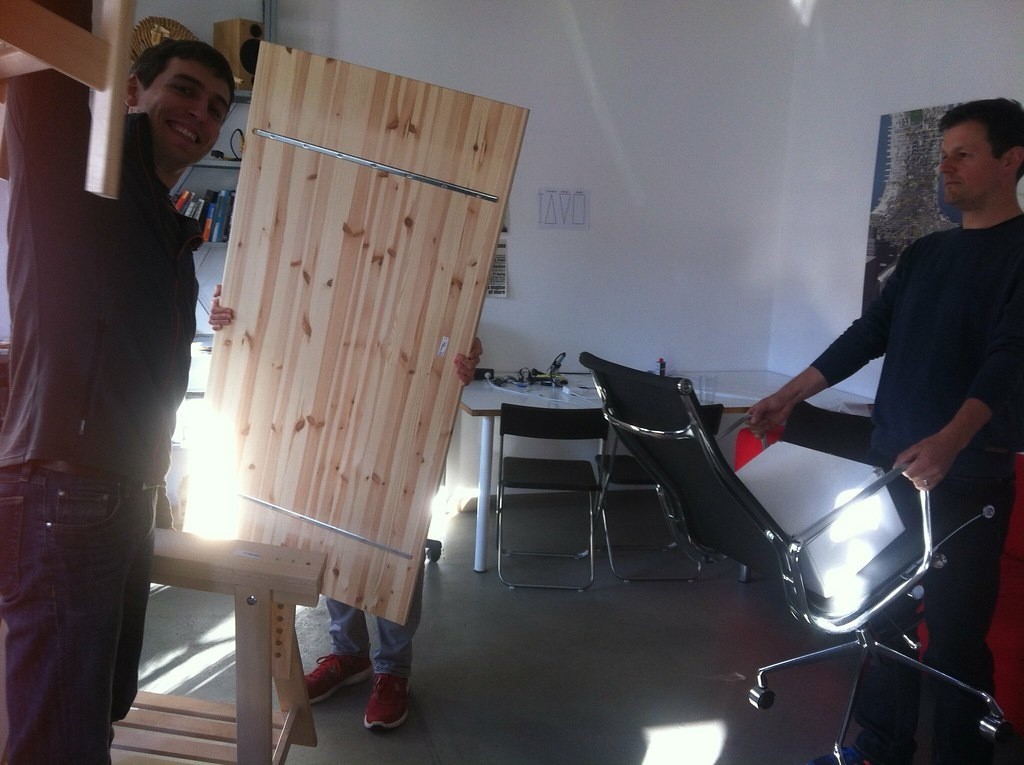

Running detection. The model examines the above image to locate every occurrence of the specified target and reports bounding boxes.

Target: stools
[109,527,328,764]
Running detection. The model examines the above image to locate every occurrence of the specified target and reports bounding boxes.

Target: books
[170,190,237,242]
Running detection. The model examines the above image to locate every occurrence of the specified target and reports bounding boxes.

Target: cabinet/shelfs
[166,90,254,343]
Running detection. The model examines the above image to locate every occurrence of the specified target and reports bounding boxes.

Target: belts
[32,462,134,488]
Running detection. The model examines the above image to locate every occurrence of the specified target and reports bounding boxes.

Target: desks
[458,369,876,574]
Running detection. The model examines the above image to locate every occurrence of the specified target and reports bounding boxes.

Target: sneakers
[305,651,374,705]
[364,673,411,730]
[815,744,869,765]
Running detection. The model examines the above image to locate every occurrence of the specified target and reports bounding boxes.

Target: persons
[208,284,484,730]
[745,97,1024,765]
[0,0,237,765]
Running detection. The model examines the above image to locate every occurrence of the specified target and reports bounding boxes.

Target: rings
[924,479,928,486]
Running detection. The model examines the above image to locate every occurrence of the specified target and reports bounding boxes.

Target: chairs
[593,405,724,583]
[496,403,608,593]
[578,351,1013,765]
